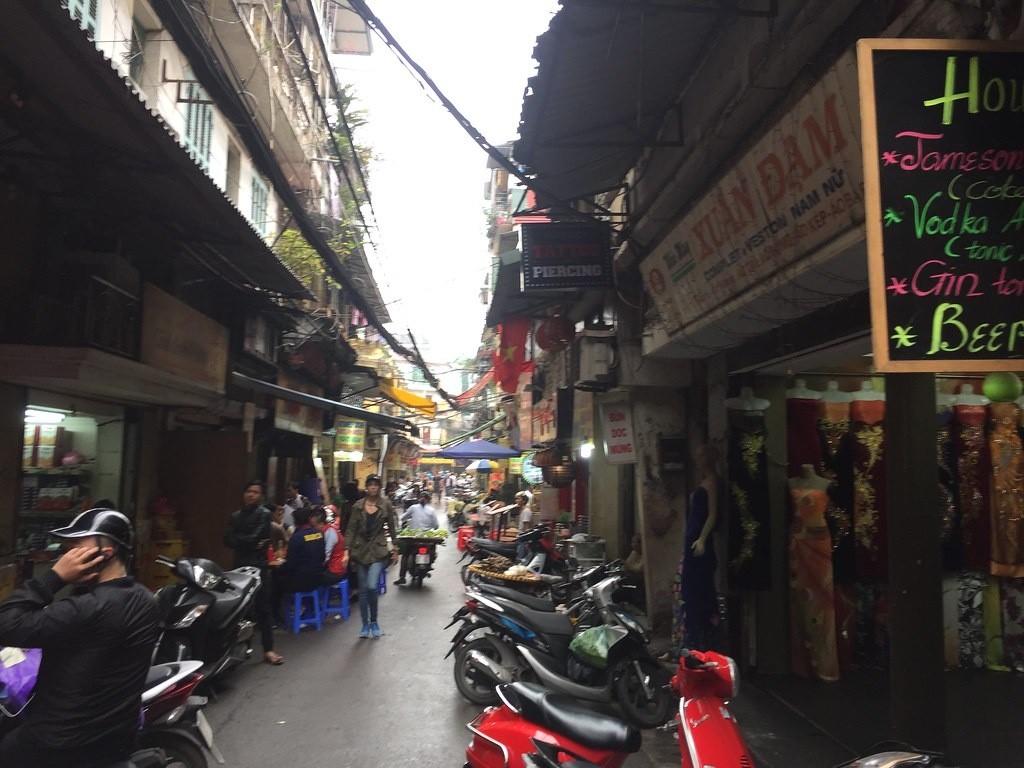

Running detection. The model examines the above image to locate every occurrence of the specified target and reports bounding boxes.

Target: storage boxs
[38,426,66,446]
[23,425,38,445]
[35,486,74,510]
[146,536,192,577]
[148,575,180,591]
[22,445,34,467]
[36,445,64,467]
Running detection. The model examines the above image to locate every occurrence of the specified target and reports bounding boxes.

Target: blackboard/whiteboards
[857,39,1024,372]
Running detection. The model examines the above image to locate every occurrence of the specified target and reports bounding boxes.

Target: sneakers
[360,625,371,637]
[370,622,381,638]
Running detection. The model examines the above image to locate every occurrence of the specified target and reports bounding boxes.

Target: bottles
[277,541,283,550]
[268,544,274,561]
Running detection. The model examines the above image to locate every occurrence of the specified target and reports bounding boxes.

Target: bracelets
[392,548,399,552]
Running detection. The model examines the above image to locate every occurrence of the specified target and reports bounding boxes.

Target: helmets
[48,508,134,551]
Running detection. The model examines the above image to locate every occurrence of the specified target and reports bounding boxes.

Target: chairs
[457,524,475,548]
[457,529,475,552]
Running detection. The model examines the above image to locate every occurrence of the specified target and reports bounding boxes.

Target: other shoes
[264,651,284,664]
[394,578,406,584]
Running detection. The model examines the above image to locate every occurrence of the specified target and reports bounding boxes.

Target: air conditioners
[569,338,617,388]
[368,433,387,450]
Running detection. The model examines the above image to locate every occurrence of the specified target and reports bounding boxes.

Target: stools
[377,567,388,595]
[283,591,323,637]
[323,577,352,620]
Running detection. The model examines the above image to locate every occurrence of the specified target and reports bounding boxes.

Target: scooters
[402,481,678,730]
[148,553,264,704]
[458,646,757,768]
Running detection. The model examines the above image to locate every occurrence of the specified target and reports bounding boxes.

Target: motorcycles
[393,518,445,589]
[0,646,226,768]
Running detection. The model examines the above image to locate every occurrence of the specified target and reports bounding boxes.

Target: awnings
[361,375,438,423]
[228,368,422,441]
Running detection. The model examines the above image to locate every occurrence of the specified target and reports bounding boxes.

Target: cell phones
[83,552,109,576]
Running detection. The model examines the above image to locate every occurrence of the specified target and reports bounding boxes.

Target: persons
[340,473,400,640]
[0,500,166,768]
[265,482,349,629]
[514,488,535,559]
[394,498,439,584]
[615,530,647,614]
[396,468,470,514]
[222,479,283,666]
[662,444,730,659]
[326,477,368,533]
[786,462,840,696]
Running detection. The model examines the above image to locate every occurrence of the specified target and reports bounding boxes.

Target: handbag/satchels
[568,623,608,668]
[0,646,42,716]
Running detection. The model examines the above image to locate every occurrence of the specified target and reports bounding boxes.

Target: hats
[366,474,381,484]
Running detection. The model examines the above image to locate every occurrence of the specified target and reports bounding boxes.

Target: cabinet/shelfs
[18,461,94,550]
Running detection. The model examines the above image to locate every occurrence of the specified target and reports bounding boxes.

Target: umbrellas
[435,436,521,492]
[466,458,499,491]
